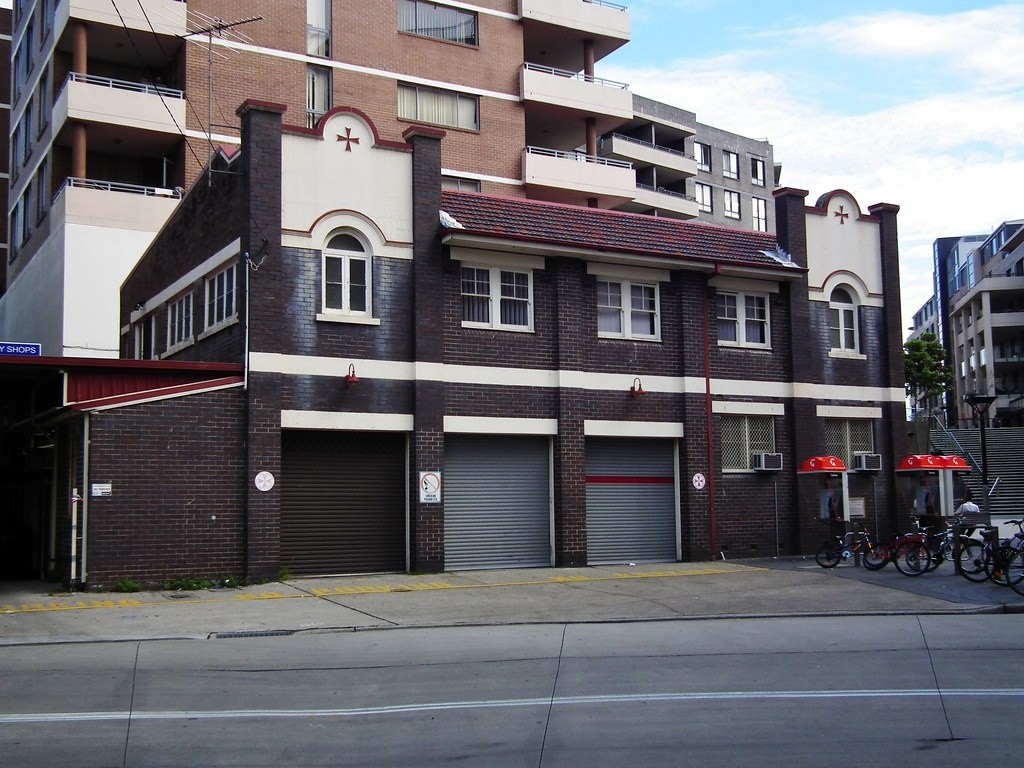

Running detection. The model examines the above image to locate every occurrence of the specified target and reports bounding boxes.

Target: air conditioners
[854,454,882,470]
[753,453,782,470]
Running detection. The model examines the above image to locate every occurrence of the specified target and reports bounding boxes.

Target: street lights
[964,392,999,517]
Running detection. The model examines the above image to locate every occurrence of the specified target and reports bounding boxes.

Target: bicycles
[815,522,890,571]
[863,532,930,577]
[905,522,991,574]
[955,517,1024,596]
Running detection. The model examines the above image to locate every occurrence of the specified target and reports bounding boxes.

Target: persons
[924,491,935,514]
[828,497,842,548]
[991,415,1001,428]
[954,496,980,537]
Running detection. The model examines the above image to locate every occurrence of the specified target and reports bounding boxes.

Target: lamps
[344,363,360,386]
[630,377,646,397]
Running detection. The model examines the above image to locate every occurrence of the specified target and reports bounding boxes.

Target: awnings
[895,455,972,471]
[797,455,848,474]
